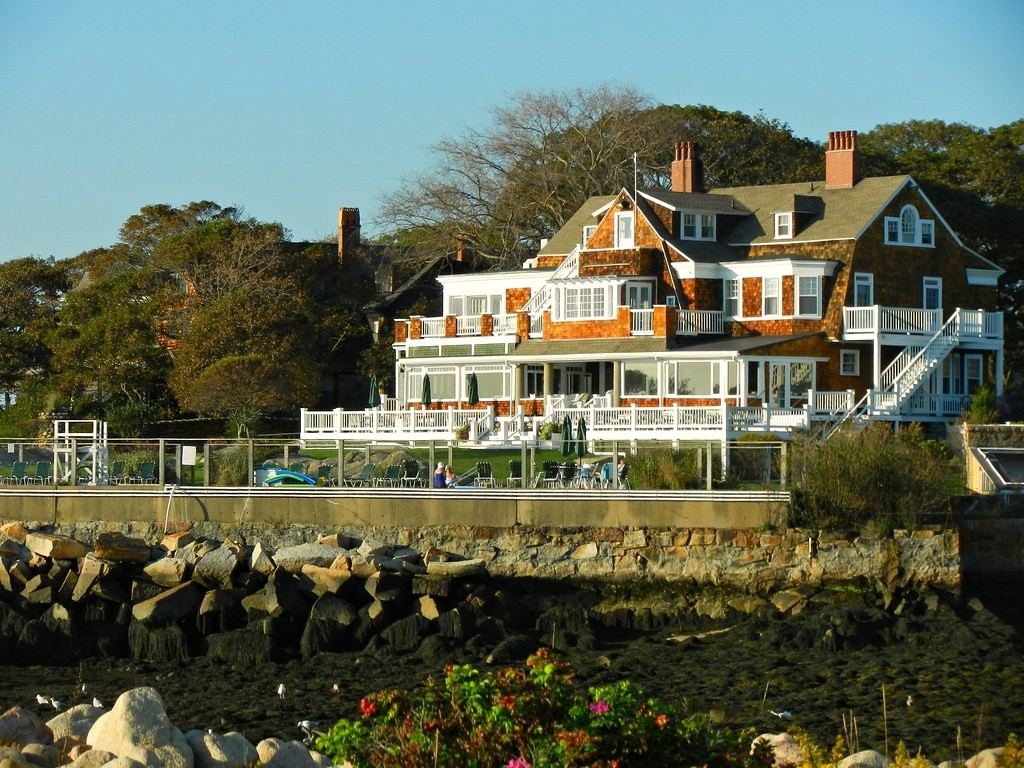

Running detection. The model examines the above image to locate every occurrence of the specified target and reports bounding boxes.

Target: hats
[438,462,444,469]
[445,465,451,470]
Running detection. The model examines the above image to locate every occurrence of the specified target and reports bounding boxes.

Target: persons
[445,465,458,487]
[616,458,627,483]
[435,462,449,488]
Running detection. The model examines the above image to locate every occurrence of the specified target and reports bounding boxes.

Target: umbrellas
[368,373,381,408]
[419,373,431,410]
[468,371,480,409]
[558,415,576,463]
[573,418,588,468]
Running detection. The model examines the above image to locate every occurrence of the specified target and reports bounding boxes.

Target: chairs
[109,460,128,484]
[316,464,335,488]
[372,465,402,488]
[29,461,52,485]
[331,463,376,487]
[5,461,29,485]
[418,462,429,487]
[129,462,157,484]
[474,461,498,488]
[507,459,522,489]
[530,460,632,490]
[401,459,423,488]
